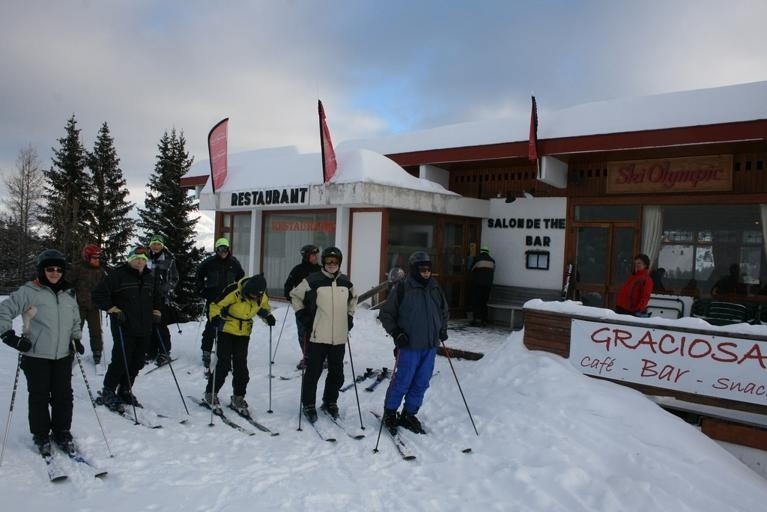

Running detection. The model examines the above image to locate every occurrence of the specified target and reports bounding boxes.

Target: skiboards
[44,452,107,482]
[311,411,364,440]
[341,367,391,392]
[93,390,189,429]
[357,280,387,310]
[369,409,471,462]
[560,263,577,302]
[71,348,107,375]
[186,394,279,436]
[138,355,177,378]
[269,357,348,380]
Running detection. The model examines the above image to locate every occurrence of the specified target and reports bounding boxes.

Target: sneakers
[34,434,53,458]
[401,408,421,432]
[202,350,211,362]
[322,401,339,417]
[52,432,76,457]
[297,357,330,370]
[100,388,126,414]
[117,387,141,405]
[155,355,172,366]
[383,410,399,431]
[303,404,319,424]
[202,390,222,410]
[92,350,102,364]
[229,394,250,414]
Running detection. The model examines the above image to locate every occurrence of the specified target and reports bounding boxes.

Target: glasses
[46,265,64,272]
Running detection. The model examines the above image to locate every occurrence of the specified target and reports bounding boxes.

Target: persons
[195,237,245,362]
[206,273,275,412]
[283,245,330,368]
[443,243,467,307]
[616,253,653,316]
[386,267,404,289]
[145,234,181,364]
[289,246,357,421]
[95,247,163,410]
[0,250,85,459]
[380,251,449,432]
[69,244,107,359]
[461,245,495,326]
[652,262,748,300]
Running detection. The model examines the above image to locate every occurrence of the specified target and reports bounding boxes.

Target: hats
[214,238,230,252]
[245,272,267,294]
[127,234,165,262]
[480,245,489,253]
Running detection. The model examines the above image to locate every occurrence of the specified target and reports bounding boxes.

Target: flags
[319,101,340,182]
[527,97,541,159]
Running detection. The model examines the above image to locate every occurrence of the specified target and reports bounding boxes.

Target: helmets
[388,250,432,282]
[36,247,66,268]
[299,243,344,273]
[81,243,102,264]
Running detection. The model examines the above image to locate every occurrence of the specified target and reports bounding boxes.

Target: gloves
[390,326,411,346]
[0,329,33,353]
[265,314,276,326]
[210,315,225,331]
[439,327,448,342]
[69,338,84,355]
[151,309,163,325]
[108,305,126,324]
